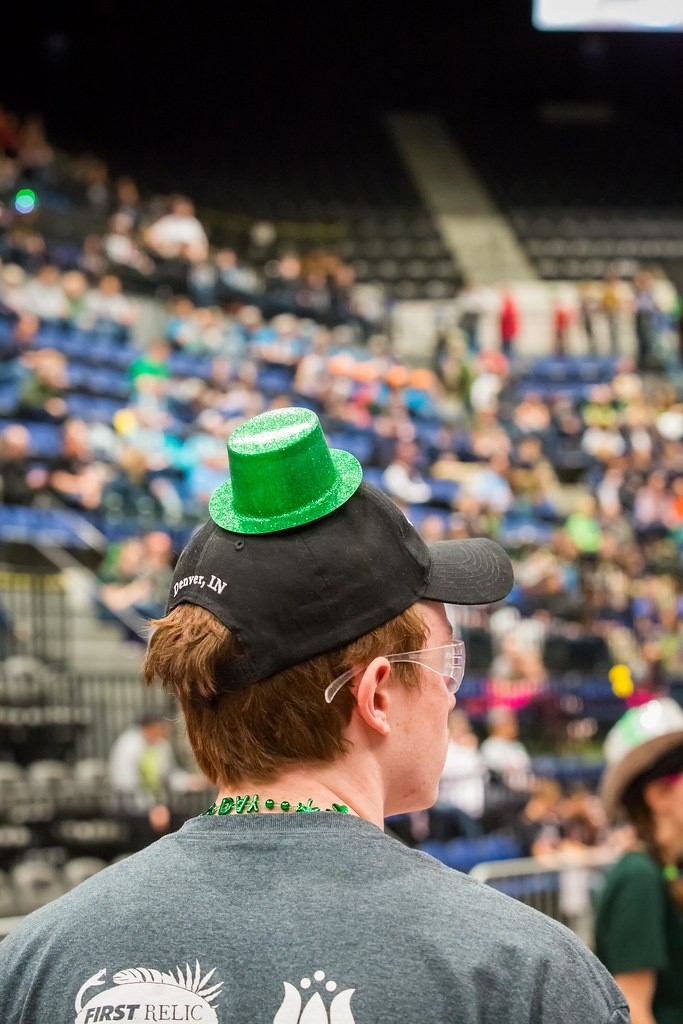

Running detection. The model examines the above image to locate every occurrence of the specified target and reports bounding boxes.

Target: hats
[164,480,515,700]
[600,696,683,830]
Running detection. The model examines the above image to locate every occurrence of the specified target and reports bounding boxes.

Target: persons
[3,116,682,944]
[593,698,683,1023]
[4,406,632,1023]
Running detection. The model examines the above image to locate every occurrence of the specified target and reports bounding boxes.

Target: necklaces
[200,794,348,815]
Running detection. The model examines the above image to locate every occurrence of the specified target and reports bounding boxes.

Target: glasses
[324,638,469,707]
